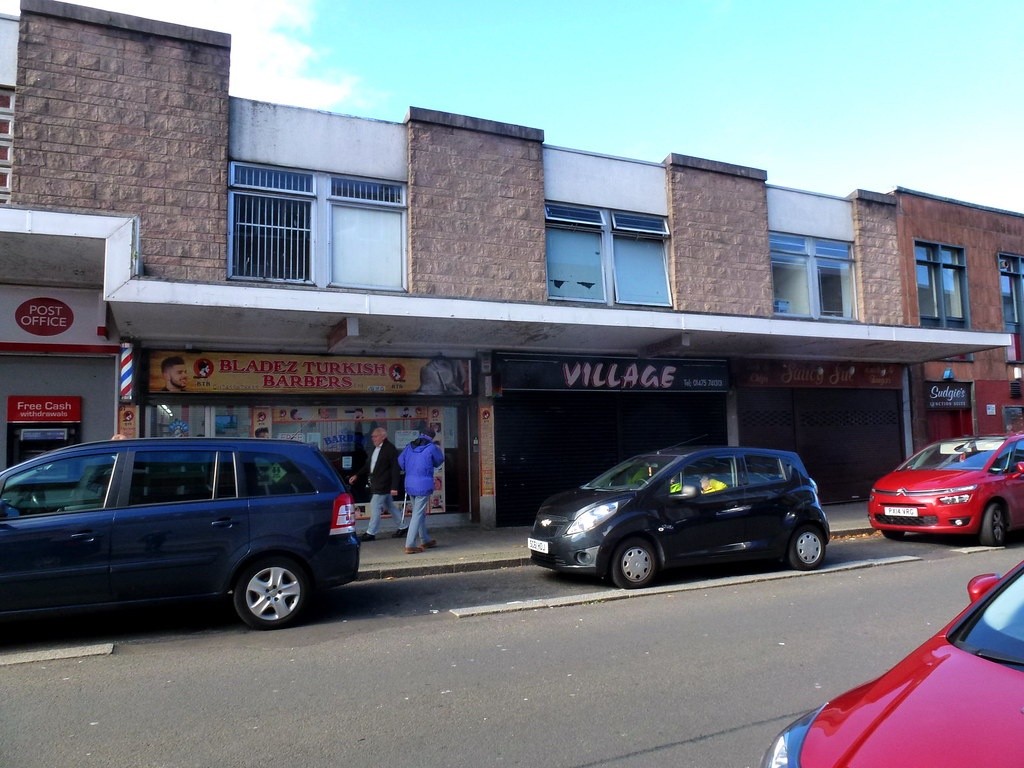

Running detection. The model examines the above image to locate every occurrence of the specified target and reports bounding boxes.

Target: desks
[775,299,792,313]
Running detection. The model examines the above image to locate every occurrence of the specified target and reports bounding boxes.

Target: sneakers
[357,533,375,542]
[392,527,410,537]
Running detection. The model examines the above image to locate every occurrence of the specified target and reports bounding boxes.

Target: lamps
[816,363,886,376]
[942,368,954,379]
[1014,367,1022,379]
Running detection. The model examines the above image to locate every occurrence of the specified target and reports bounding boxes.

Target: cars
[527,445,830,589]
[868,431,1024,547]
[1,436,361,631]
[758,558,1024,768]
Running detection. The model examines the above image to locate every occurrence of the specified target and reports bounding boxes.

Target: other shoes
[421,540,437,548]
[405,546,422,553]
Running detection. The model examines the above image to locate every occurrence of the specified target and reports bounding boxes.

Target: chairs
[67,461,103,511]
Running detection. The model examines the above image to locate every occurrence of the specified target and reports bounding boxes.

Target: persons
[669,465,734,498]
[415,359,466,393]
[349,428,409,538]
[87,434,126,498]
[160,357,188,393]
[254,407,441,438]
[398,427,444,551]
[400,440,444,512]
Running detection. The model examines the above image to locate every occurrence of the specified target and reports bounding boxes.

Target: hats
[421,428,436,439]
